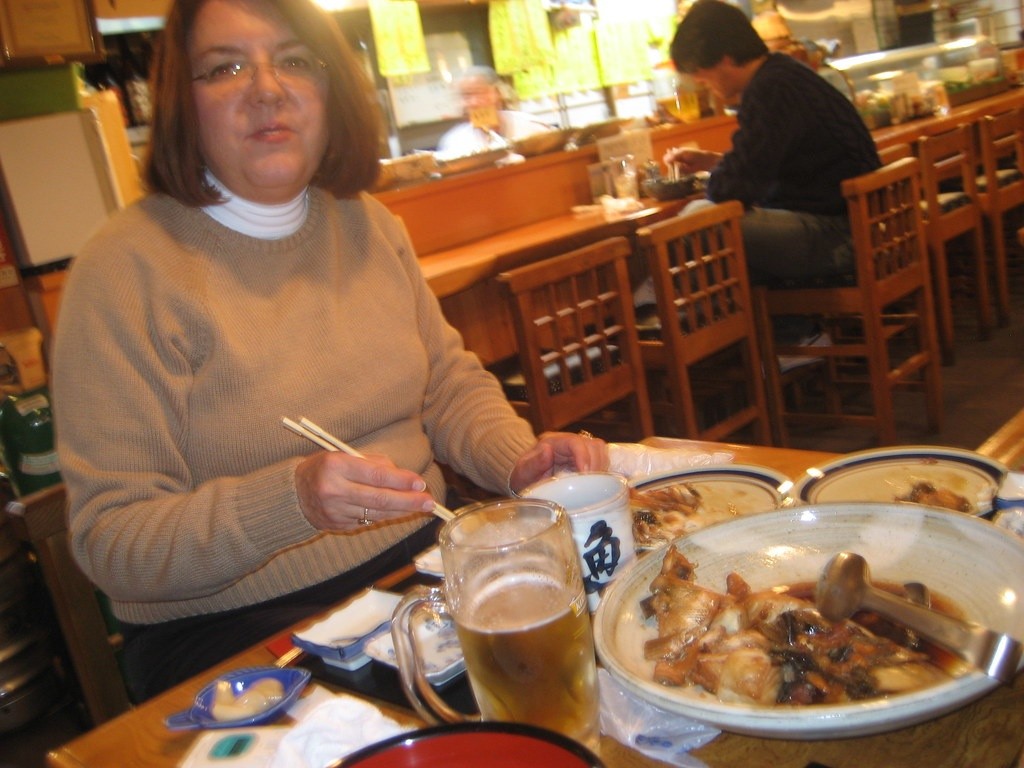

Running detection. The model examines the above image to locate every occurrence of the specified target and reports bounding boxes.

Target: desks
[45,436,1024,768]
[415,87,1024,366]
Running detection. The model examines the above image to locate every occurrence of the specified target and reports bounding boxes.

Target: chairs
[500,236,656,444]
[600,199,777,449]
[903,122,1000,345]
[954,106,1024,315]
[757,156,949,446]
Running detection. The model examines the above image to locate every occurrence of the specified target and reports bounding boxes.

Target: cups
[609,153,639,201]
[389,499,602,758]
[514,470,636,610]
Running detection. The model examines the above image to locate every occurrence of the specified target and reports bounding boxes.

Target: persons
[633,0,882,372]
[436,67,553,155]
[48,0,608,696]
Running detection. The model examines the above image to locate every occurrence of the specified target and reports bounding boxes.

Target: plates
[159,666,311,730]
[412,542,445,580]
[289,586,402,670]
[436,149,509,173]
[362,615,466,686]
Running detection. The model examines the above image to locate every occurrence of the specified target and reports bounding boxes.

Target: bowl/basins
[793,444,1012,523]
[640,176,695,200]
[326,720,605,767]
[592,500,1024,740]
[625,464,793,555]
[510,129,575,159]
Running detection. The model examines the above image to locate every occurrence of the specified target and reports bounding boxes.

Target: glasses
[192,54,331,88]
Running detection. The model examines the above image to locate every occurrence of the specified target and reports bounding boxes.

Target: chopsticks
[281,417,455,522]
[666,147,680,182]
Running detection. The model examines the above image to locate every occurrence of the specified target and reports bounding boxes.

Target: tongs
[815,550,1024,688]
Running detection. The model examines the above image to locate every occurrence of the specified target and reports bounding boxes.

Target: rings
[359,508,372,526]
[578,429,593,439]
